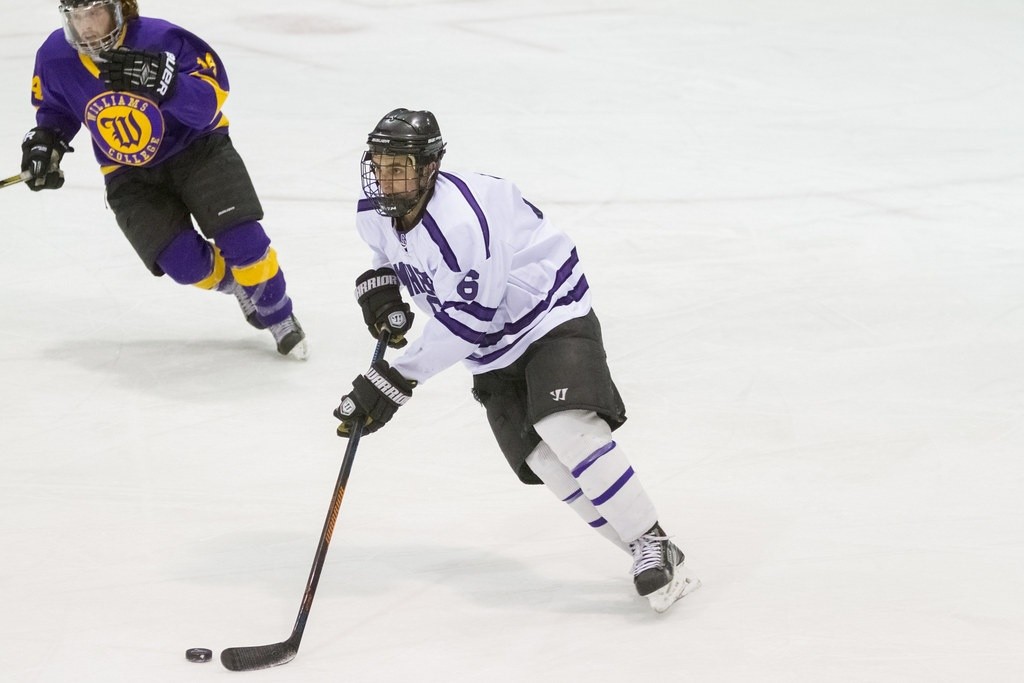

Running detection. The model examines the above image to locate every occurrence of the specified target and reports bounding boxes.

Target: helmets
[360,108,447,218]
[60,1,138,61]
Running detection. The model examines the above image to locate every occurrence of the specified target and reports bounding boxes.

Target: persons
[20,0,311,364]
[333,107,703,614]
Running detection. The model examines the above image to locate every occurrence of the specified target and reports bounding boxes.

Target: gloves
[333,360,414,438]
[99,44,175,104]
[21,128,75,192]
[355,268,416,348]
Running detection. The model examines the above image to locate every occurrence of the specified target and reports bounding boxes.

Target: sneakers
[269,315,311,359]
[627,522,701,614]
[234,283,267,330]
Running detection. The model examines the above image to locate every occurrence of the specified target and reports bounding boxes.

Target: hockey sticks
[0,171,32,191]
[219,319,391,676]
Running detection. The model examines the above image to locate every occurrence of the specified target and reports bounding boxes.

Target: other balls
[186,646,213,663]
